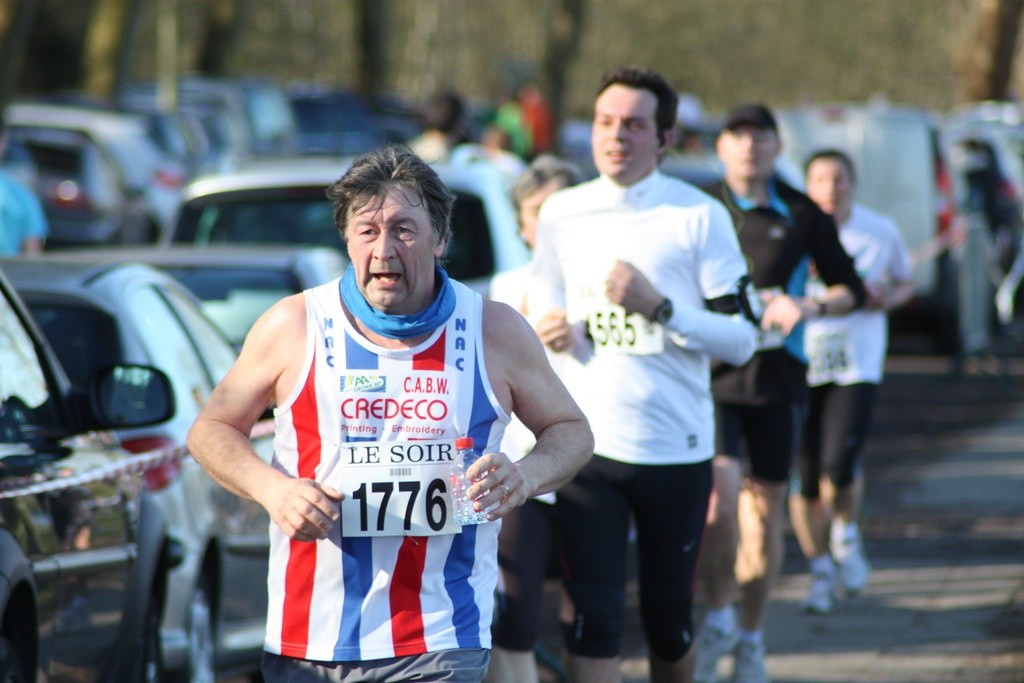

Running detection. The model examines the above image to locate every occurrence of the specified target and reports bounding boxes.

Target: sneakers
[839,555,871,593]
[735,642,766,683]
[692,629,731,683]
[804,580,838,614]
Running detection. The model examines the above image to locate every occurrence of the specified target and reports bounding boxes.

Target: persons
[0,93,48,258]
[185,143,596,683]
[407,64,915,682]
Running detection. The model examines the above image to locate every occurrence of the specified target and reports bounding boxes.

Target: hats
[726,103,776,131]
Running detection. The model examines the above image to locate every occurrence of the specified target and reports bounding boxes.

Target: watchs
[813,296,828,317]
[651,298,673,324]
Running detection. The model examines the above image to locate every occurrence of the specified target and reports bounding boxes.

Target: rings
[501,484,509,498]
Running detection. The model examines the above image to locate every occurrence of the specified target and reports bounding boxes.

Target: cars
[0,75,1024,683]
